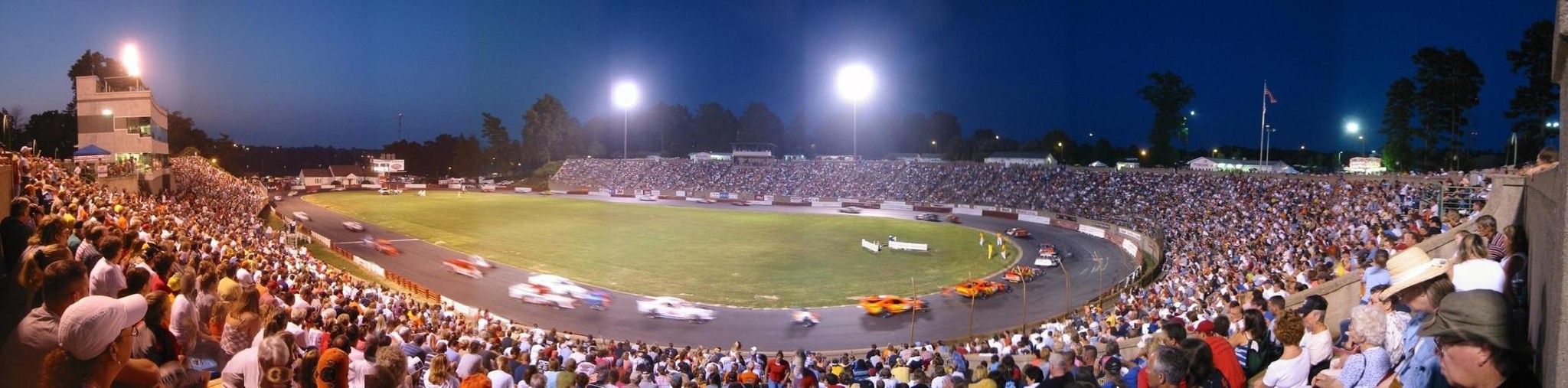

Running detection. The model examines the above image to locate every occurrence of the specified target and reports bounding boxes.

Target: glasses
[120,326,139,338]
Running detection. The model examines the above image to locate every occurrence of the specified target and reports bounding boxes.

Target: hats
[1379,247,1452,302]
[316,348,349,388]
[290,308,308,321]
[1294,294,1328,313]
[58,293,147,361]
[67,203,77,211]
[21,146,32,153]
[241,261,249,266]
[1416,289,1536,355]
[1187,319,1215,334]
[167,273,182,291]
[266,280,278,290]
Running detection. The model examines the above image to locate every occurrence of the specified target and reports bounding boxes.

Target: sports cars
[273,186,404,256]
[639,194,657,202]
[1005,226,1031,238]
[539,190,554,196]
[696,199,716,205]
[946,214,962,224]
[916,211,940,222]
[479,188,494,193]
[838,207,861,214]
[731,200,753,207]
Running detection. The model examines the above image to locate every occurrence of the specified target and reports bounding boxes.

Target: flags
[1266,88,1278,104]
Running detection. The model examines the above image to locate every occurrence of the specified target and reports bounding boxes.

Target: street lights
[931,139,939,153]
[1359,134,1365,157]
[1211,149,1217,158]
[1058,141,1064,160]
[1338,150,1343,164]
[611,80,643,160]
[834,63,882,158]
[1545,121,1559,146]
[398,112,403,140]
[1265,124,1276,173]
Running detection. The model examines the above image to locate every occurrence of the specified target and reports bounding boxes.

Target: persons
[0,148,1556,388]
